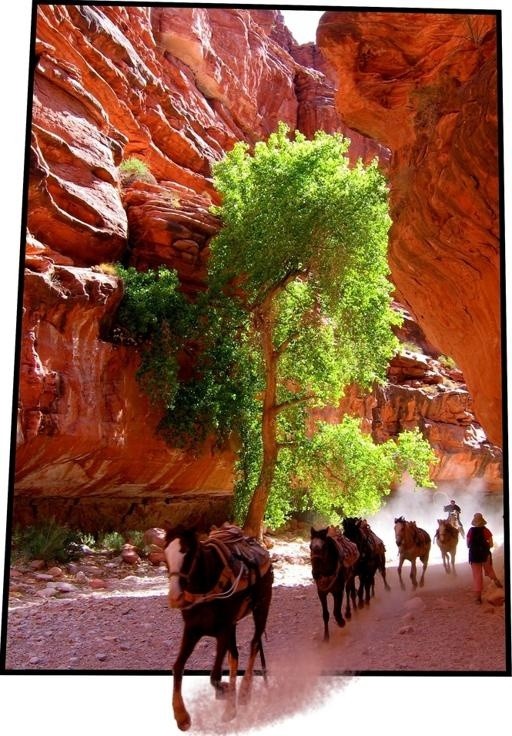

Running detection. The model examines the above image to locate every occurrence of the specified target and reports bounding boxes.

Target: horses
[436,519,458,576]
[449,509,459,530]
[162,521,274,732]
[394,515,431,592]
[340,516,391,610]
[309,526,358,644]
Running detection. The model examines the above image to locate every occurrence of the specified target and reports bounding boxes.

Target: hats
[472,513,486,526]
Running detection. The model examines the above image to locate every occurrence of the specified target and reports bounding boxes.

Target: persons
[440,500,465,533]
[465,512,504,605]
